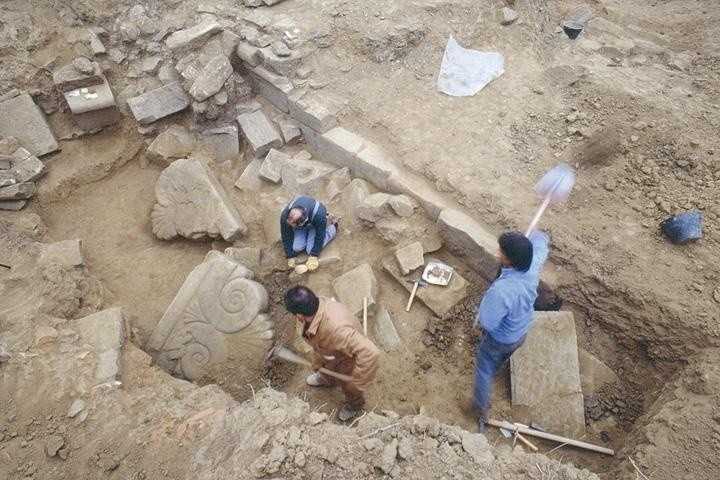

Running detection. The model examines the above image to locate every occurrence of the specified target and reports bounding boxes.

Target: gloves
[305,256,319,271]
[288,257,295,268]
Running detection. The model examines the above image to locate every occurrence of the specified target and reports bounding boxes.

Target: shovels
[499,164,576,278]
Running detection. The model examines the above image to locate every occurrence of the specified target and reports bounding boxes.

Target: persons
[279,195,339,275]
[457,223,550,426]
[283,285,381,423]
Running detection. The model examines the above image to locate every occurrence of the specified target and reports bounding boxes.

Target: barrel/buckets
[660,212,703,245]
[564,21,584,40]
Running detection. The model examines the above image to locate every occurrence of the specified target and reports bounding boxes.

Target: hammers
[405,279,429,312]
[512,427,539,452]
[479,416,614,457]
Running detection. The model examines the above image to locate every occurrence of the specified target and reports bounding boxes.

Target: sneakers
[340,403,356,421]
[332,219,338,232]
[307,372,336,386]
